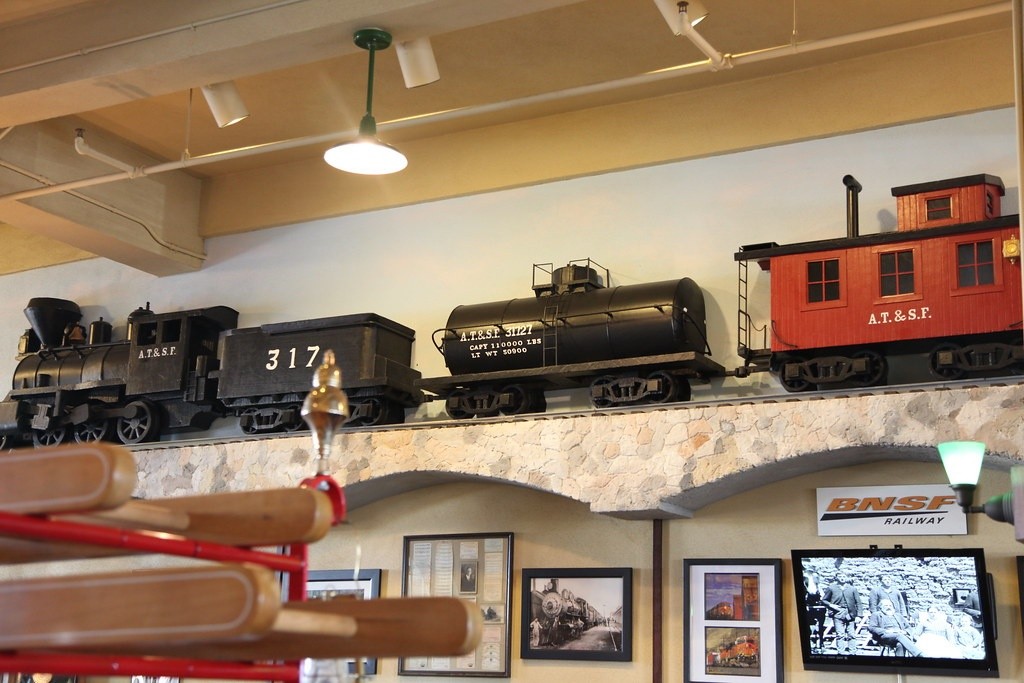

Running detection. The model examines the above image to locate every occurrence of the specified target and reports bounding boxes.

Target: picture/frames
[682,557,784,683]
[307,569,382,675]
[520,568,633,661]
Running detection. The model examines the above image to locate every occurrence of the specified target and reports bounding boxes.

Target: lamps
[395,36,441,89]
[652,0,726,67]
[201,81,250,129]
[323,29,408,175]
[936,439,1024,543]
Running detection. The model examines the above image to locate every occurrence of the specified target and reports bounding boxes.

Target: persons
[822,573,957,656]
[575,618,584,640]
[530,618,543,646]
[461,567,475,592]
[602,617,610,626]
[553,617,561,639]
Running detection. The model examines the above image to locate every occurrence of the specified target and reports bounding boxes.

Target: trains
[530,578,604,649]
[1,171,1024,450]
[706,601,731,620]
[718,635,758,668]
